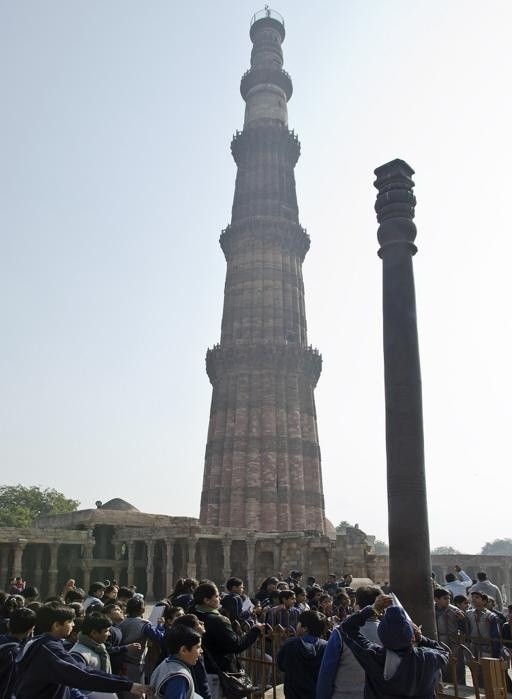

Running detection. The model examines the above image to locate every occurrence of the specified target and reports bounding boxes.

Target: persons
[3,558,511,698]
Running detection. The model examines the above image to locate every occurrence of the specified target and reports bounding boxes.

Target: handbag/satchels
[221,669,253,699]
